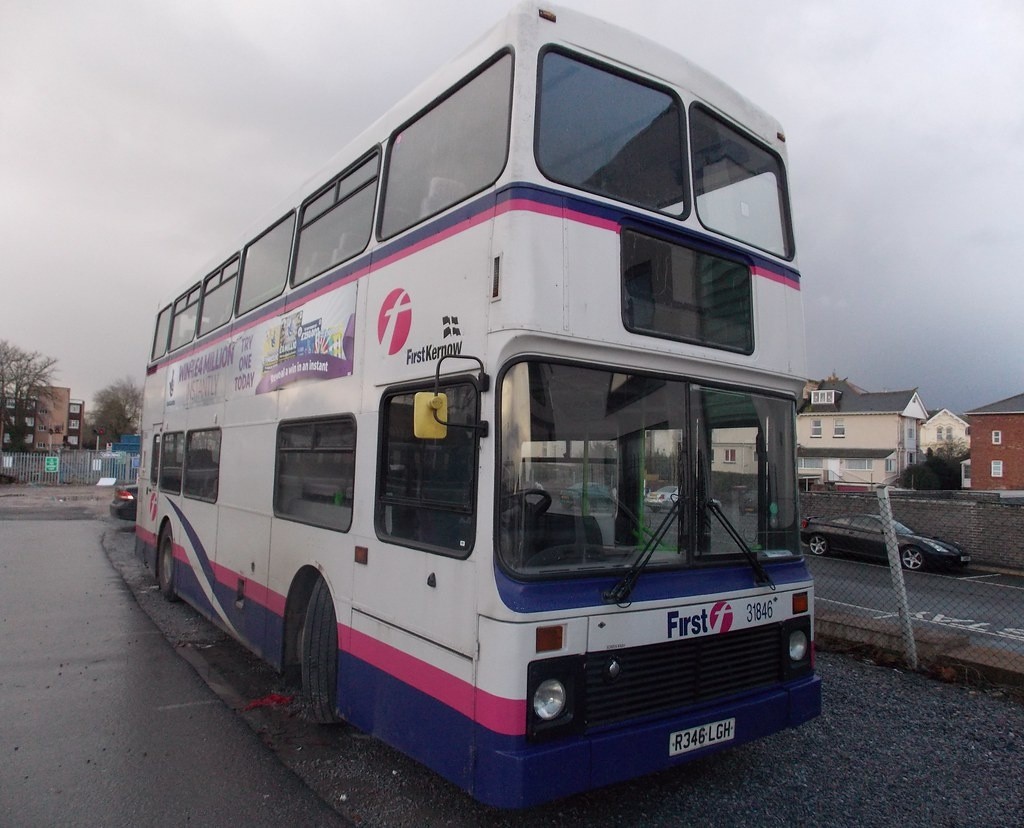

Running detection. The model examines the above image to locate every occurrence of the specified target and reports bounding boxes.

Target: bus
[134,2,825,807]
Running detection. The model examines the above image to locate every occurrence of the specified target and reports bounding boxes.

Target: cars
[800,512,971,575]
[561,482,615,509]
[739,489,760,517]
[110,486,140,522]
[644,486,722,515]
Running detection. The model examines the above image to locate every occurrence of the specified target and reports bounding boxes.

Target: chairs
[419,175,466,218]
[184,449,217,486]
[181,322,209,345]
[305,233,365,281]
[545,512,603,549]
